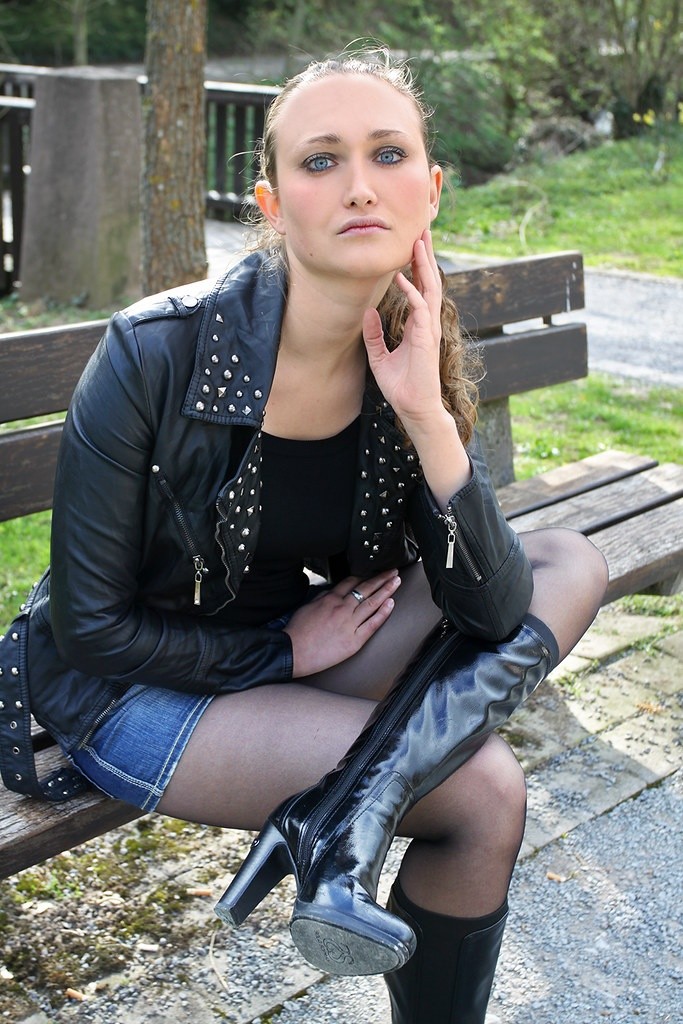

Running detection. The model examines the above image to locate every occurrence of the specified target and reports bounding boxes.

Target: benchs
[1,252,683,879]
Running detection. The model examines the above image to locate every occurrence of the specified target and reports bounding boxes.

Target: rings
[351,590,364,603]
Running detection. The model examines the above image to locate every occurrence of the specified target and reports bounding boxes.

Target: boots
[214,610,561,975]
[375,890,510,1024]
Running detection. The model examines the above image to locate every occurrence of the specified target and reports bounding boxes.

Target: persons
[0,59,611,1023]
